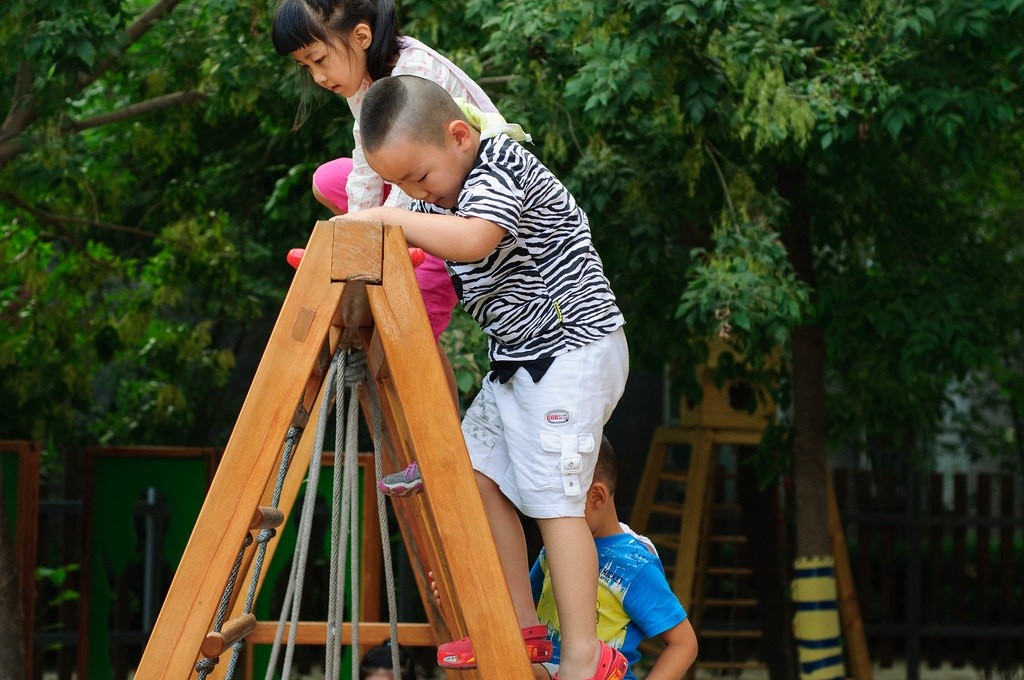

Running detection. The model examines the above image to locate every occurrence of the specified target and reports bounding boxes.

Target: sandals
[553,639,628,680]
[436,623,554,670]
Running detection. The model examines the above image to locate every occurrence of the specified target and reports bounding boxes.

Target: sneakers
[379,460,424,497]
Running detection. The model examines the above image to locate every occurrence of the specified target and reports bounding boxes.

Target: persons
[358,633,415,680]
[267,0,523,498]
[330,74,630,680]
[528,434,698,680]
